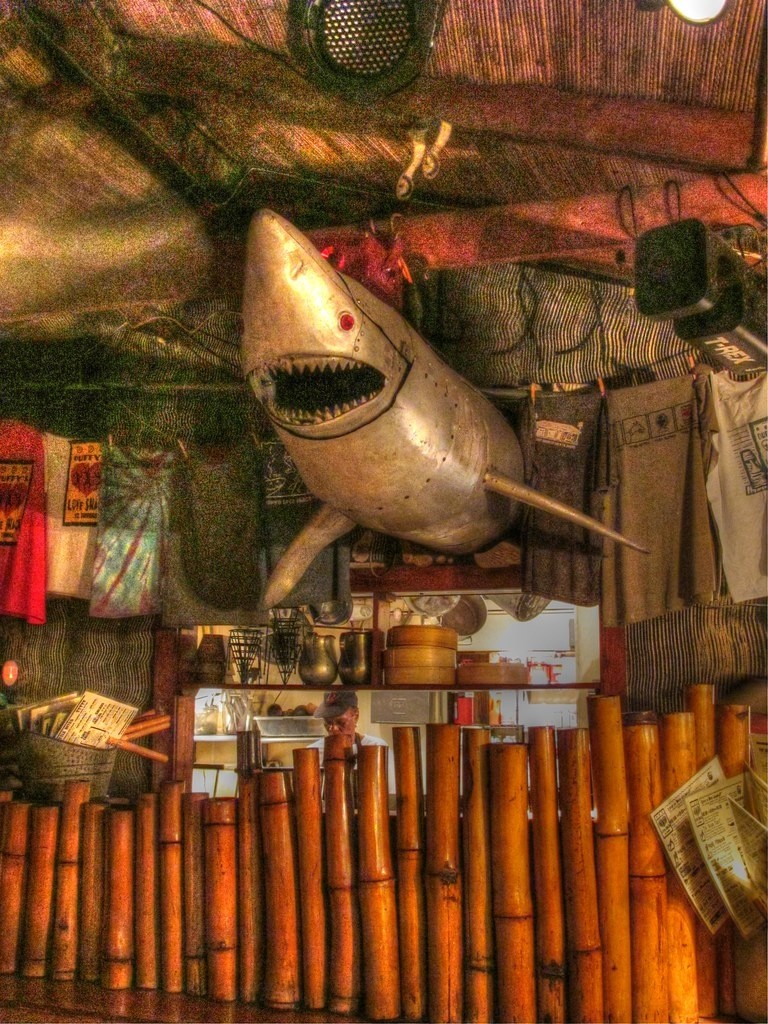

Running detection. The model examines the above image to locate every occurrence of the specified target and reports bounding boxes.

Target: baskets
[13,727,117,804]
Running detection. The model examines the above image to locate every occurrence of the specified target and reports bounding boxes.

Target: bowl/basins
[192,732,238,770]
[248,716,326,737]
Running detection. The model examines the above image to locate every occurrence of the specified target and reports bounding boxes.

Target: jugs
[527,655,552,685]
[339,629,371,685]
[298,632,337,684]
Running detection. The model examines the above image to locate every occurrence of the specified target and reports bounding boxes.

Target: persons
[306,691,396,795]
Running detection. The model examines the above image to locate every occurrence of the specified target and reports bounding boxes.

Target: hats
[314,691,359,717]
